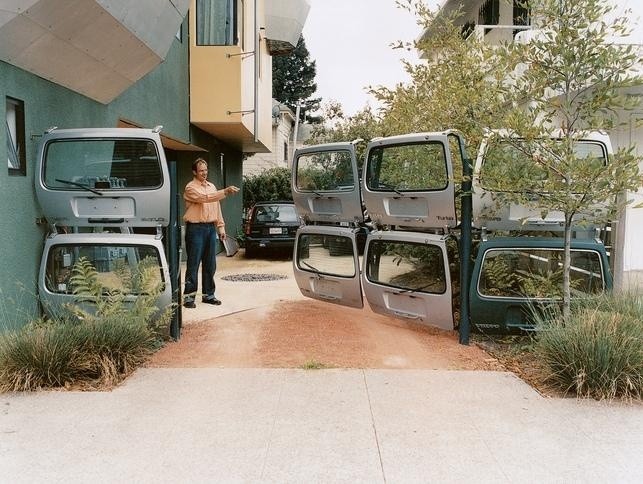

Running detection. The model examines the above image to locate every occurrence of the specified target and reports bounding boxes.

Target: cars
[243,200,309,259]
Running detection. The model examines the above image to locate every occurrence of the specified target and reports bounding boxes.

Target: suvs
[322,185,388,256]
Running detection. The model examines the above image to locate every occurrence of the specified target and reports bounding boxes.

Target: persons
[183,158,241,309]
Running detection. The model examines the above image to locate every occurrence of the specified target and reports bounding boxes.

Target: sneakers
[202,296,221,305]
[183,301,196,308]
